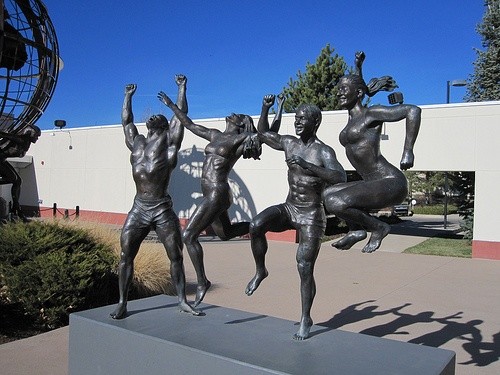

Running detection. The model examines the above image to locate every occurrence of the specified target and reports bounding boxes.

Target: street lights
[443,80,467,229]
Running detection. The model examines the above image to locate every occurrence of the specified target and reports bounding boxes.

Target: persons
[320,50,423,255]
[155,89,285,309]
[247,94,346,341]
[109,74,202,320]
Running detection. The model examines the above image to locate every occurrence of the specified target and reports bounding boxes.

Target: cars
[390,200,415,216]
